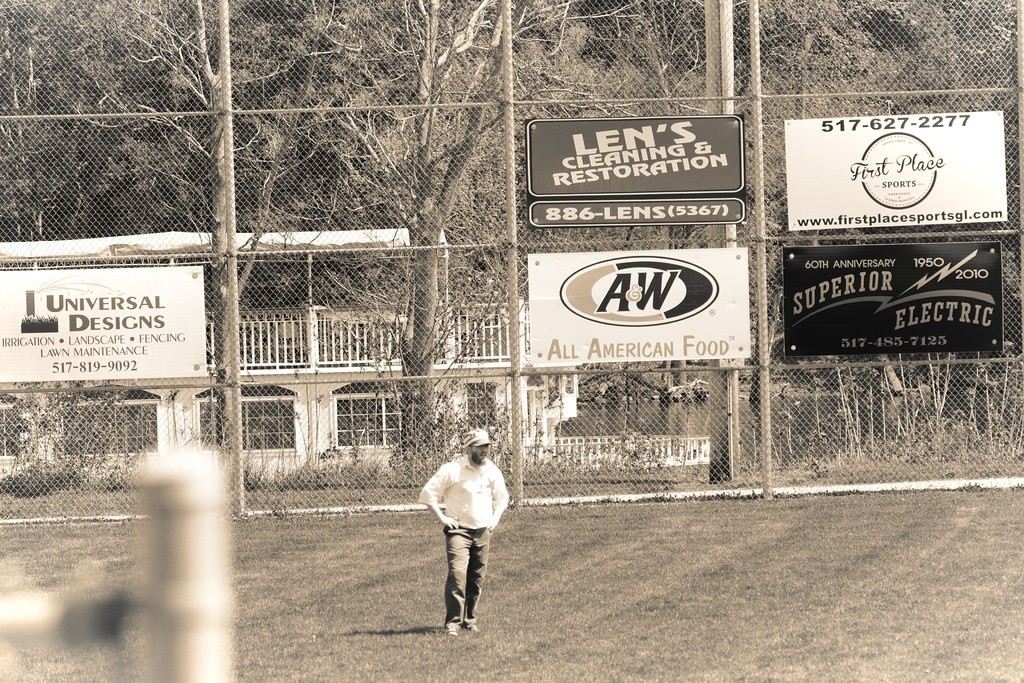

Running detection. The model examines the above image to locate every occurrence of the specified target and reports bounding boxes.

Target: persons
[419,429,509,635]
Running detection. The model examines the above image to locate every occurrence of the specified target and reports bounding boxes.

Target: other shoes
[461,623,477,630]
[447,627,458,635]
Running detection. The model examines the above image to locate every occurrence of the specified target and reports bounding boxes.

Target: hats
[464,430,495,446]
